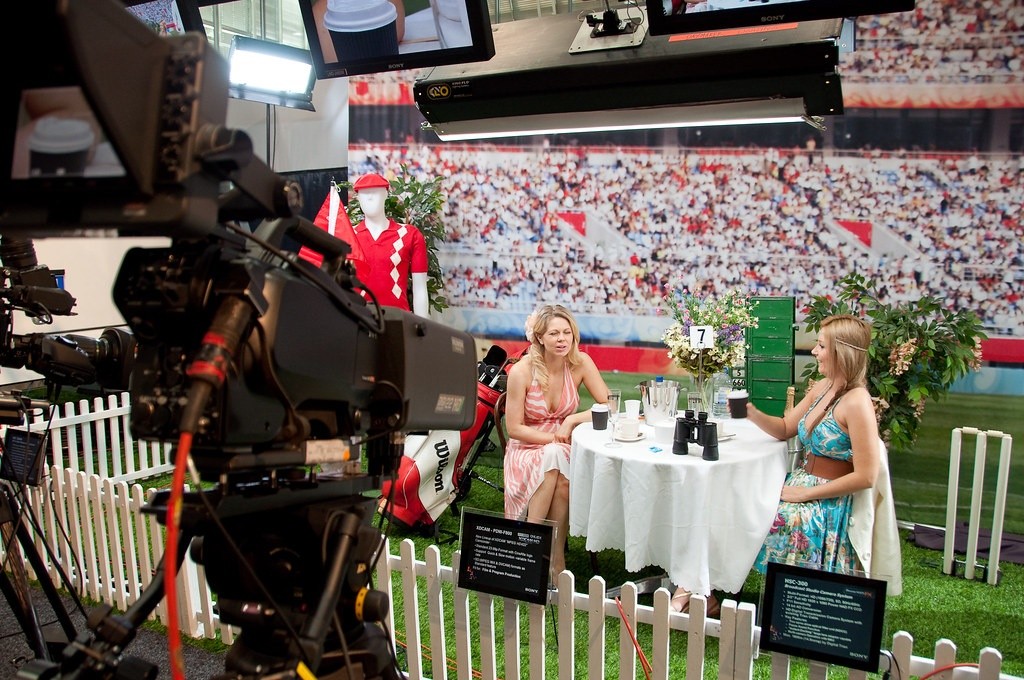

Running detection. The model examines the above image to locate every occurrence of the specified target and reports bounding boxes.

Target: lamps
[227,34,317,170]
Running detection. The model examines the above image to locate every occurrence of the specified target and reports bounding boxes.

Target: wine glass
[606,389,621,447]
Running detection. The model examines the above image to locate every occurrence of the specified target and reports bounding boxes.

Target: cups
[324,2,398,66]
[591,403,608,430]
[708,419,724,435]
[688,391,701,419]
[728,389,749,419]
[28,122,92,176]
[624,401,640,423]
[621,420,638,439]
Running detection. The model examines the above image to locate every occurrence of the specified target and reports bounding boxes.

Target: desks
[567,410,788,598]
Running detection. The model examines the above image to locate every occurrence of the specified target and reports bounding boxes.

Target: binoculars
[671,409,719,461]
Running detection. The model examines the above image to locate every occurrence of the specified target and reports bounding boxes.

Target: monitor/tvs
[298,0,495,80]
[125,0,209,41]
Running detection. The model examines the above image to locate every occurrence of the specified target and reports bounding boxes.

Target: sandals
[670,590,720,619]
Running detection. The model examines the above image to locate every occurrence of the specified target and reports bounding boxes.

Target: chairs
[494,391,508,455]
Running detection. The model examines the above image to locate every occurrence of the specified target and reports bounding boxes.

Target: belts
[802,453,854,480]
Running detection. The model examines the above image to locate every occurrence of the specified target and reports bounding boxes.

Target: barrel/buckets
[635,379,681,421]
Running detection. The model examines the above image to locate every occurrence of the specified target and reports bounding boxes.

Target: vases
[689,371,714,417]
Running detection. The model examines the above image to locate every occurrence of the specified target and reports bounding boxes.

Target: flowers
[800,269,990,455]
[657,282,761,379]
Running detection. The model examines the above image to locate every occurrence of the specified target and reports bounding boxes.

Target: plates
[717,437,730,441]
[615,435,645,441]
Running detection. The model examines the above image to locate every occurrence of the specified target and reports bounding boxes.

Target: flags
[331,182,367,263]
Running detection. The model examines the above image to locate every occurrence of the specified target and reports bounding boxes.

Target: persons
[503,305,616,588]
[670,314,878,618]
[353,173,429,320]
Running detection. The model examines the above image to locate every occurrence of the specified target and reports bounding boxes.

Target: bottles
[654,376,664,388]
[713,367,732,418]
